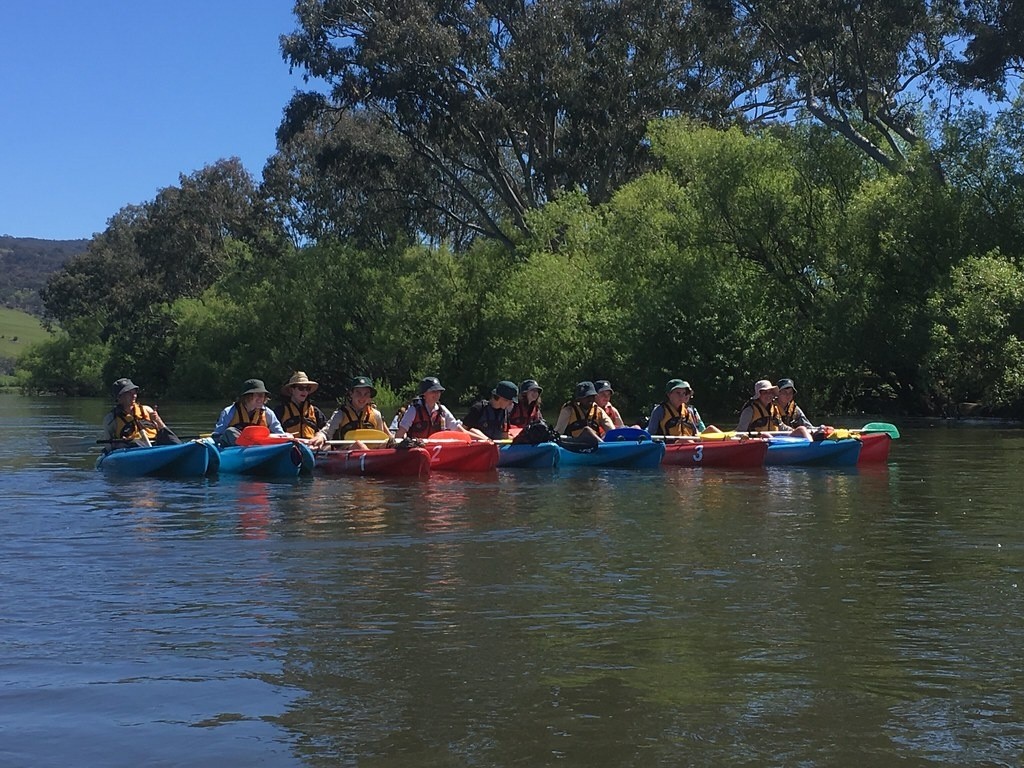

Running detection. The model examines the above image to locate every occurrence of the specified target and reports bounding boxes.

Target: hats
[574,381,597,399]
[665,379,685,393]
[520,379,543,396]
[749,380,779,400]
[112,378,139,403]
[280,371,319,395]
[235,379,271,400]
[777,379,797,396]
[348,376,377,400]
[491,381,518,404]
[594,380,614,397]
[683,381,694,396]
[412,393,422,400]
[417,377,445,397]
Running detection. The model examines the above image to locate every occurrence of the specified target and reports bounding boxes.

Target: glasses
[493,395,500,401]
[686,389,690,395]
[292,385,311,391]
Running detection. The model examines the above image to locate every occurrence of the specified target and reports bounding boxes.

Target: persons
[462,381,518,446]
[735,380,814,442]
[682,382,723,433]
[505,380,543,427]
[309,377,396,450]
[388,403,414,434]
[103,378,183,452]
[212,379,294,446]
[553,382,615,442]
[774,379,827,431]
[395,377,494,446]
[648,379,749,443]
[275,372,328,444]
[594,380,640,429]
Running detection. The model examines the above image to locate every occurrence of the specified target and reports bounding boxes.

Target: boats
[556,438,666,470]
[219,442,315,476]
[95,436,220,477]
[767,441,863,465]
[661,440,769,471]
[309,445,431,477]
[497,443,560,468]
[857,434,892,461]
[421,439,500,471]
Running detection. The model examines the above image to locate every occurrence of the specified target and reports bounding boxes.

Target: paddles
[344,428,573,445]
[96,433,222,444]
[700,422,900,438]
[237,424,472,446]
[605,428,701,441]
